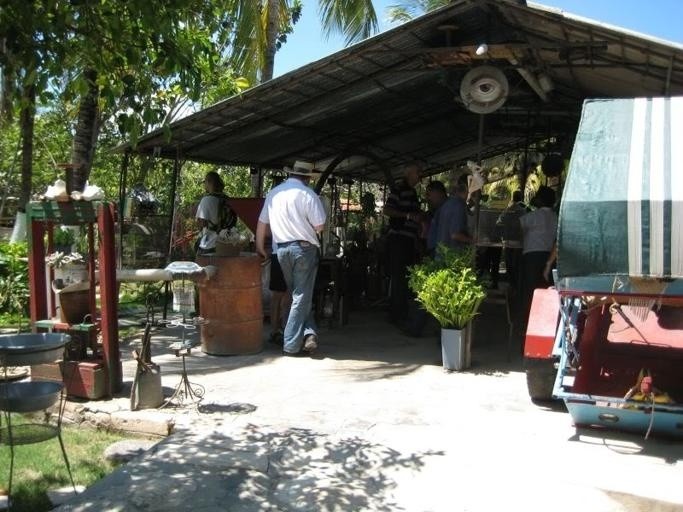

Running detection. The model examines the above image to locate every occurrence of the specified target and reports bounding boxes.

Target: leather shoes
[282,351,311,356]
[301,335,317,354]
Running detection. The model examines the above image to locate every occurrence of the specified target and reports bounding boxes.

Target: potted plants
[418,268,488,371]
[405,240,487,368]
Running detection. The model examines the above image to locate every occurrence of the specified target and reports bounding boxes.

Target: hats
[282,160,323,177]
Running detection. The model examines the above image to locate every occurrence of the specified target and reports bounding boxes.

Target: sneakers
[268,329,283,346]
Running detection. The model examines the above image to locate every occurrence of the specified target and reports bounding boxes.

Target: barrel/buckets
[196,252,264,356]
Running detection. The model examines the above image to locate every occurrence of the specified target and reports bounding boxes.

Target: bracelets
[405,209,411,222]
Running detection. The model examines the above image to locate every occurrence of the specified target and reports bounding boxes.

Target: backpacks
[216,203,237,235]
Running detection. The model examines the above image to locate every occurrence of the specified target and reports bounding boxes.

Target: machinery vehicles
[523,95,682,437]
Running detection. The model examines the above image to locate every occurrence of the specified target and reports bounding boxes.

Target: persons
[187,171,226,319]
[544,180,565,281]
[254,156,326,357]
[269,175,287,347]
[396,179,449,339]
[479,196,504,277]
[495,184,559,344]
[498,190,532,287]
[381,161,431,331]
[427,172,482,272]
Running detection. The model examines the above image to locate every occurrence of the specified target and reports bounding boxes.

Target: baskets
[214,240,241,257]
